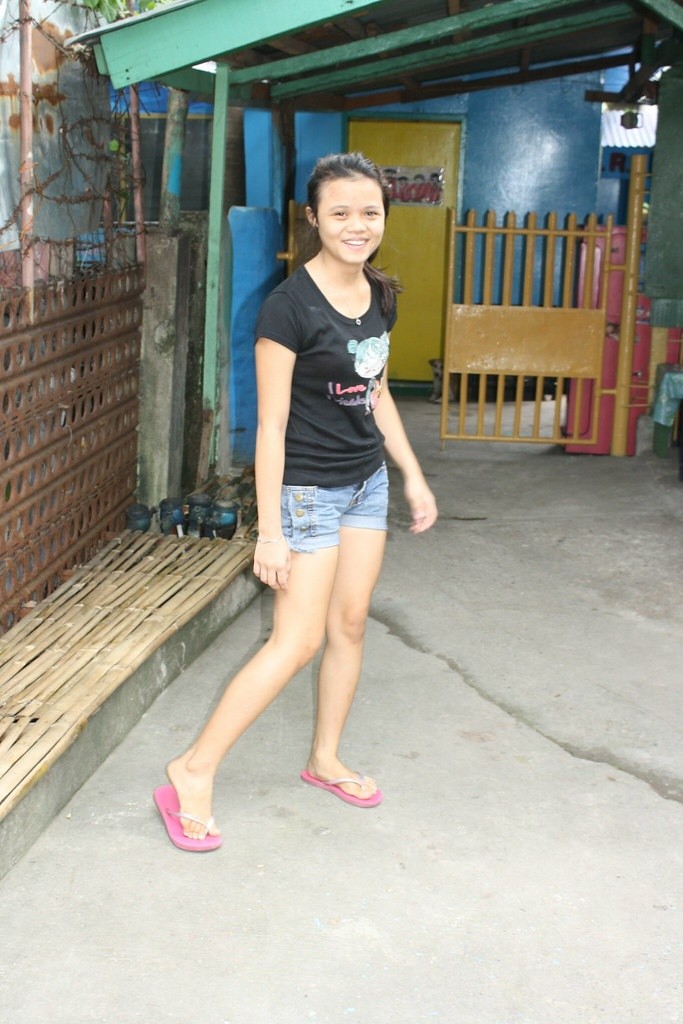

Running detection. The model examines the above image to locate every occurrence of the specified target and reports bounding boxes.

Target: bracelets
[258,534,282,543]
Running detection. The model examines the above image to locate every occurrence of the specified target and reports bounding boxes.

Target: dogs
[428,358,459,404]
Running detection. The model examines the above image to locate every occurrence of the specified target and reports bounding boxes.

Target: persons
[155,154,439,852]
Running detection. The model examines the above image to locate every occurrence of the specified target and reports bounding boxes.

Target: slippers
[155,785,222,852]
[301,767,383,808]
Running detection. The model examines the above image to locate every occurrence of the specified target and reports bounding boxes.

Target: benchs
[652,363,683,460]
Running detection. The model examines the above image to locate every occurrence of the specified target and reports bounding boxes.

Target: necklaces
[337,284,361,326]
